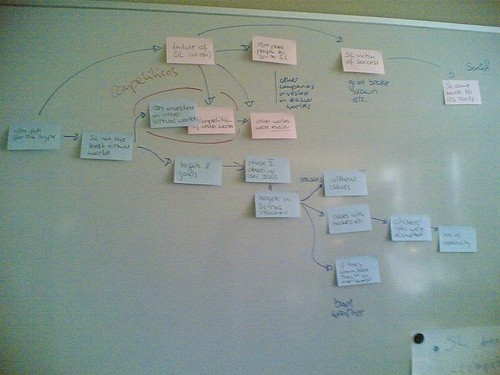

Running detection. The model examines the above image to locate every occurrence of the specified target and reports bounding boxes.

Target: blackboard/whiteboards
[1,0,500,375]
